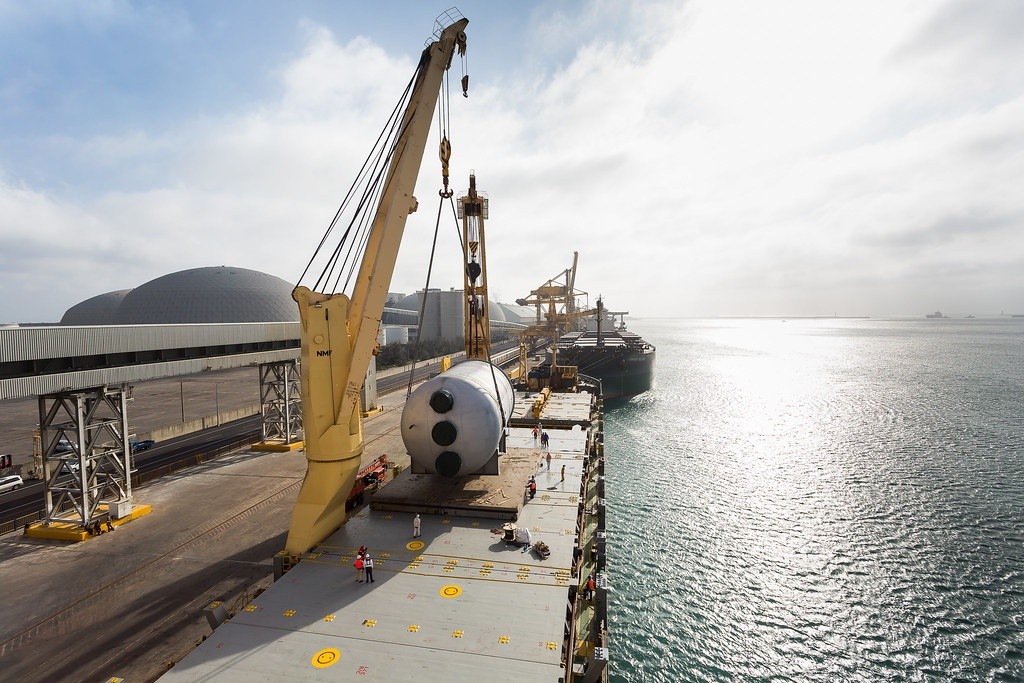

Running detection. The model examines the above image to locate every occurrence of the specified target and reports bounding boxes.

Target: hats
[366,554,370,557]
[356,555,361,559]
[416,515,419,517]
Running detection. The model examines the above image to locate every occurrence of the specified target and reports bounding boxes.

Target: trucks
[129,439,155,453]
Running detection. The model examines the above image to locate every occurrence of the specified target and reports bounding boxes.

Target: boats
[146,6,609,683]
[586,315,617,331]
[545,329,656,408]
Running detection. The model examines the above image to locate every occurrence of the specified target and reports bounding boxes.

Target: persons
[413,514,422,538]
[353,545,376,583]
[84,522,93,535]
[22,523,30,535]
[540,432,549,447]
[530,474,535,483]
[560,465,566,480]
[531,427,539,439]
[546,453,551,470]
[95,519,102,535]
[106,516,115,533]
[537,422,542,433]
[583,574,595,601]
[526,481,536,498]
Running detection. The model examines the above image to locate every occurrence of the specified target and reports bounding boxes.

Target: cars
[0,473,25,494]
[56,440,78,452]
[60,459,90,473]
